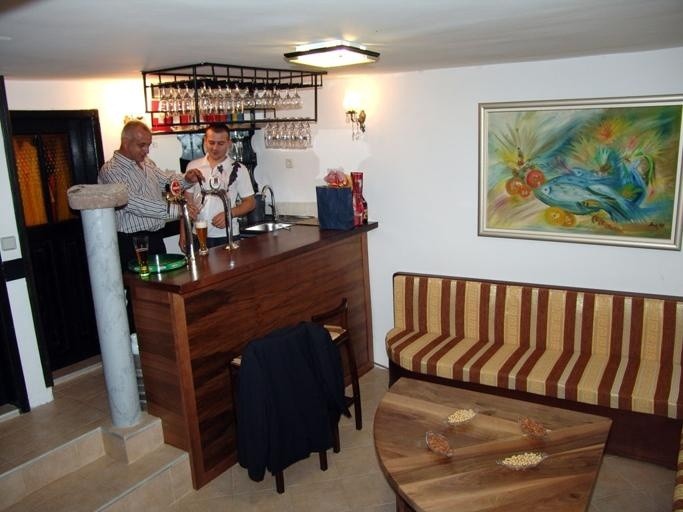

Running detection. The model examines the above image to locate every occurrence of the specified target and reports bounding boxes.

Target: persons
[96,120,205,333]
[177,123,256,253]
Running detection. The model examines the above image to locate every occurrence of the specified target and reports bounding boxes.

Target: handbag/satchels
[316,173,355,230]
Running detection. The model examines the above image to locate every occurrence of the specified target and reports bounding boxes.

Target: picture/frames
[476,94,681,252]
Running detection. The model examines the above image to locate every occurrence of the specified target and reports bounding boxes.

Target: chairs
[227,321,331,496]
[312,297,365,454]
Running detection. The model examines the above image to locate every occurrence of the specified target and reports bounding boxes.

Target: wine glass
[263,120,314,149]
[157,84,304,116]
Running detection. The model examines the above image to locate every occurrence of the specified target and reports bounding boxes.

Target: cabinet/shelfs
[140,59,328,132]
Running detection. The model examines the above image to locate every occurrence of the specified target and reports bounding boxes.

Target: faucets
[163,183,186,213]
[197,175,219,206]
[260,185,280,221]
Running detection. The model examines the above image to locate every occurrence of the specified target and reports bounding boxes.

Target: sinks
[244,221,294,233]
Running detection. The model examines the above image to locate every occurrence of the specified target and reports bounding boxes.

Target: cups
[130,231,151,281]
[192,217,209,257]
[230,140,246,165]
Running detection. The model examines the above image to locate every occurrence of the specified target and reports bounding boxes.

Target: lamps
[342,92,366,134]
[282,39,380,74]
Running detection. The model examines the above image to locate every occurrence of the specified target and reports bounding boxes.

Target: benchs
[385,269,682,471]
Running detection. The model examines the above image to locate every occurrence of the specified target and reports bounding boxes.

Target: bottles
[234,193,248,224]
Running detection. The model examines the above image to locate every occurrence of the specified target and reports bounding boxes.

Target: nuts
[428,436,449,453]
[448,409,475,423]
[520,415,546,436]
[504,452,541,467]
